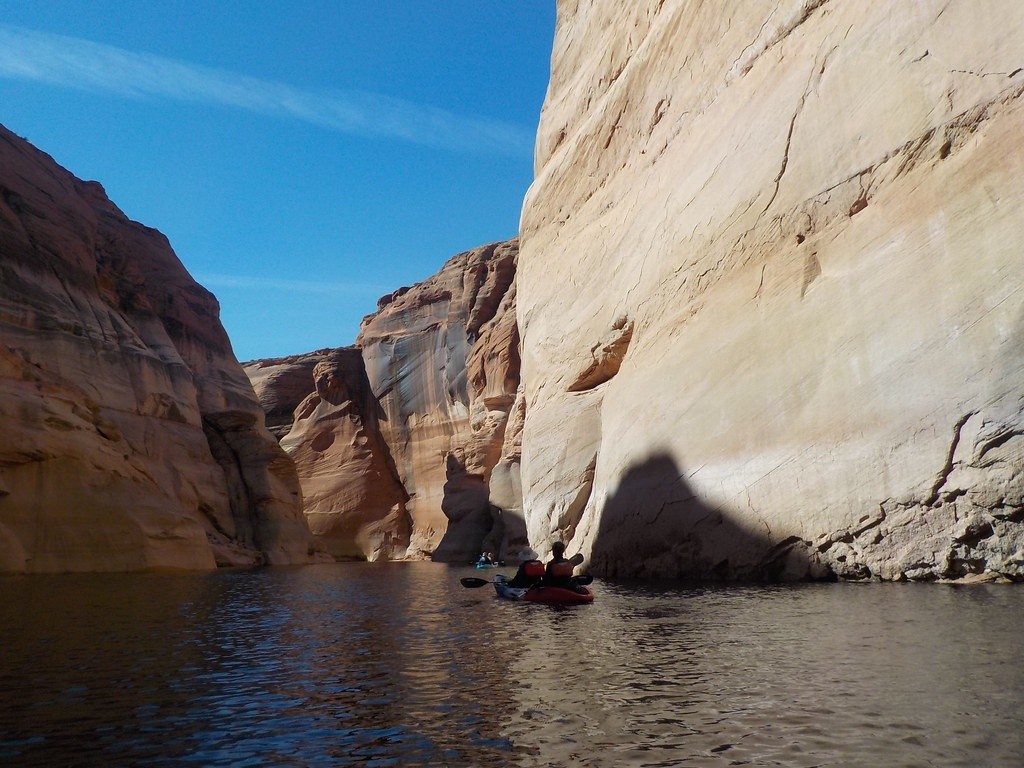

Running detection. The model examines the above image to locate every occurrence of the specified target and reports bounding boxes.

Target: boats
[494,574,595,605]
[475,562,499,570]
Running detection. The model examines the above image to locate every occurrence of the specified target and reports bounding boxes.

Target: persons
[487,553,494,565]
[544,541,574,587]
[479,552,491,565]
[507,546,545,588]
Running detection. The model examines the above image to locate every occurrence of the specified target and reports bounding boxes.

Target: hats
[483,552,486,556]
[550,542,565,552]
[519,546,539,561]
[488,553,493,557]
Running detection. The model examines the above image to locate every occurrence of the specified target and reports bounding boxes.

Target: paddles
[461,575,594,588]
[508,554,584,602]
